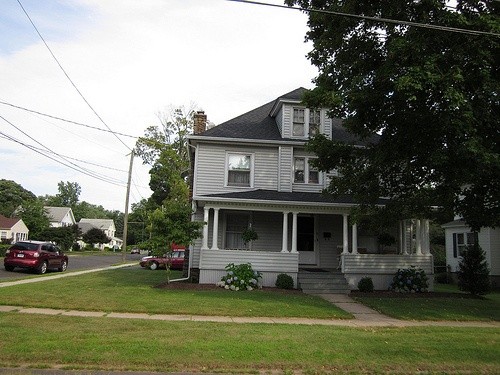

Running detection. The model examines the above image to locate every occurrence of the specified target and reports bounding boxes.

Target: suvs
[4,239,68,274]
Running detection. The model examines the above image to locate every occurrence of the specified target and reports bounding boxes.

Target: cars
[139,248,189,270]
